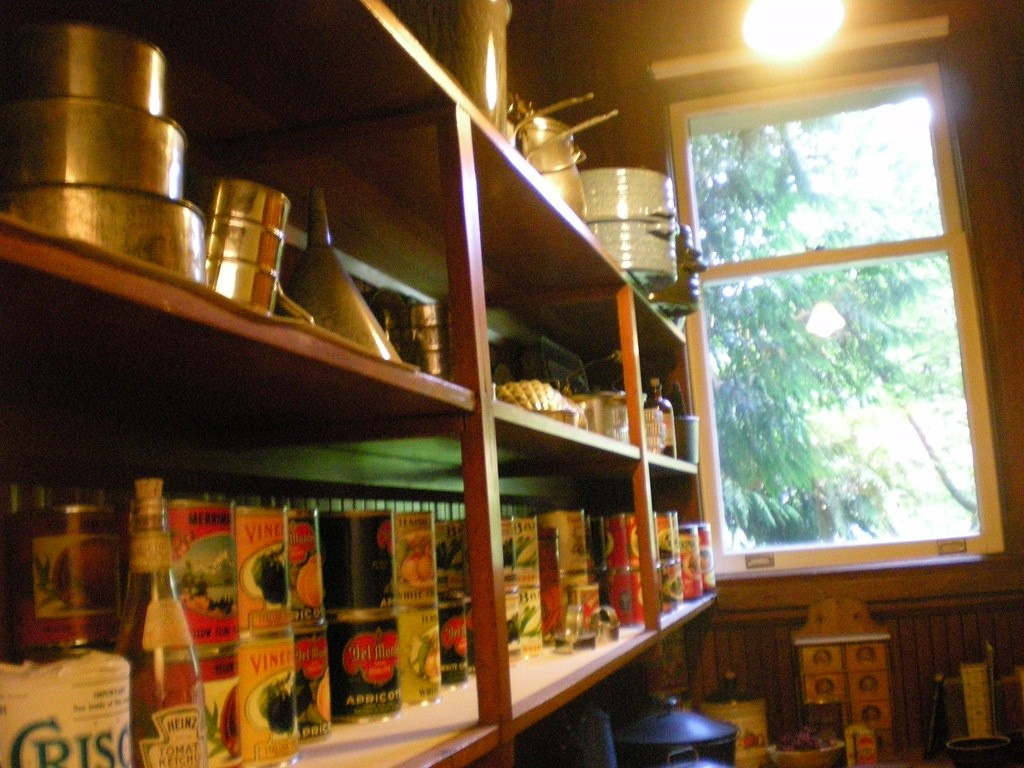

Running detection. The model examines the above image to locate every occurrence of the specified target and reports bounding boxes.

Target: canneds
[0,501,717,768]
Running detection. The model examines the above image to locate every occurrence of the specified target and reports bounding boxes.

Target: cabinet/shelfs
[0,0,719,768]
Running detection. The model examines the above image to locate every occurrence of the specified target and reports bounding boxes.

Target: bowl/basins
[17,21,167,120]
[12,97,187,201]
[7,183,207,285]
[766,739,845,768]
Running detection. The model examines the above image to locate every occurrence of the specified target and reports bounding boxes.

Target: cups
[673,415,700,465]
[513,116,587,220]
[396,0,513,138]
[948,736,1010,768]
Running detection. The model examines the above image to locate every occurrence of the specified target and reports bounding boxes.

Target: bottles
[112,477,208,768]
[644,378,677,458]
[699,671,769,768]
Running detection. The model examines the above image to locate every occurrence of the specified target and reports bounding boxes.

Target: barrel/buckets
[577,165,707,317]
[201,174,292,317]
[381,303,449,380]
